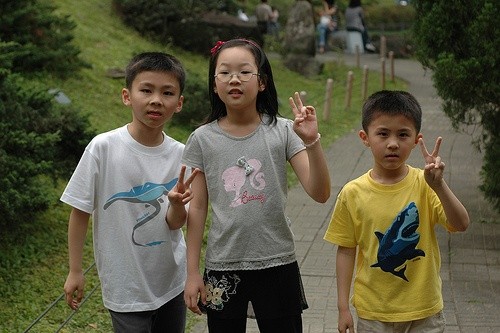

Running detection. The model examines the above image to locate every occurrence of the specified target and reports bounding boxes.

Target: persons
[344,0,376,52]
[59,52,200,333]
[323,89,470,333]
[317,0,338,53]
[237,0,280,44]
[182,39,332,333]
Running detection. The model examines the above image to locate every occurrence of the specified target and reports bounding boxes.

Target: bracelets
[302,133,321,147]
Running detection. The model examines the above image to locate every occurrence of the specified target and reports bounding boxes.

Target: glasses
[213,70,259,82]
